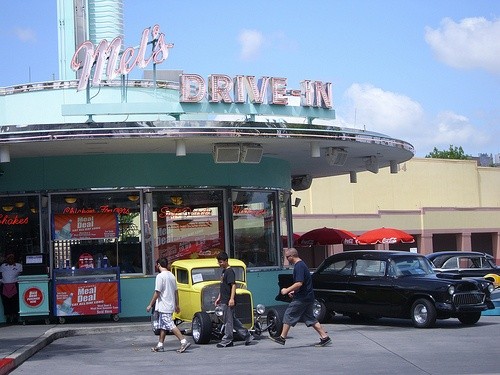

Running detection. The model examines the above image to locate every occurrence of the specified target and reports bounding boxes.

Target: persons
[269,248,330,347]
[146,257,192,353]
[214,252,254,348]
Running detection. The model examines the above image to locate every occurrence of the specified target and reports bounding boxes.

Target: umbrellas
[295,226,360,258]
[282,232,310,248]
[358,227,415,250]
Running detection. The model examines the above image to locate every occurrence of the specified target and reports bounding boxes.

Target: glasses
[286,254,293,259]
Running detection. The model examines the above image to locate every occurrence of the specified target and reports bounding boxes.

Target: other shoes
[245,335,254,346]
[217,342,234,348]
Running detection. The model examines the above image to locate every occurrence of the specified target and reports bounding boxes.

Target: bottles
[103,255,107,268]
[59,219,72,239]
[66,258,70,270]
[59,292,73,314]
[97,257,101,268]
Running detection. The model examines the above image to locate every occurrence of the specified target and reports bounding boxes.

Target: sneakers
[315,336,331,346]
[269,336,286,345]
[151,347,164,352]
[177,341,190,352]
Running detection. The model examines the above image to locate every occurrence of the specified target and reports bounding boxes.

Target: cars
[410,250,500,294]
[148,256,284,346]
[287,247,496,329]
